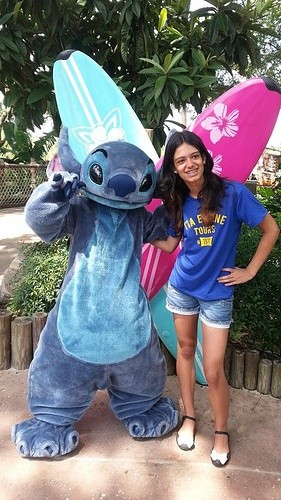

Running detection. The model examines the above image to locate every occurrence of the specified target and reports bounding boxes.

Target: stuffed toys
[10,117,179,457]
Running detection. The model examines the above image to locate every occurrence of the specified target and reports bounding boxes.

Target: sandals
[176,415,196,450]
[210,430,231,466]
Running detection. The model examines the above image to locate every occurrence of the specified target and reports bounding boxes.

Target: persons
[148,129,281,466]
